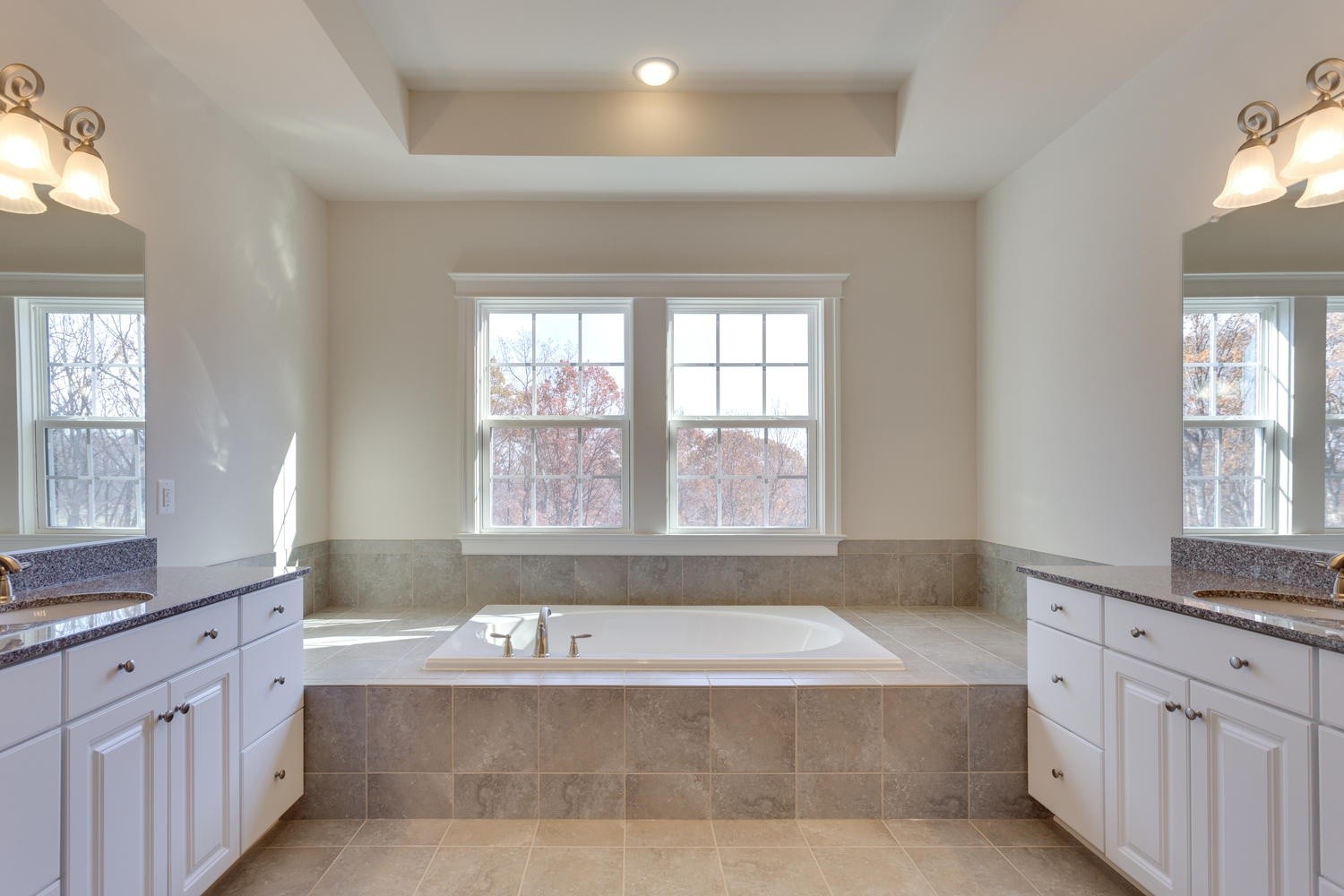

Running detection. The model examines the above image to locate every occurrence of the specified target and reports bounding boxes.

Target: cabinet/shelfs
[1027,576,1104,851]
[0,650,66,896]
[238,575,304,855]
[64,595,241,895]
[1104,595,1316,896]
[1317,648,1344,896]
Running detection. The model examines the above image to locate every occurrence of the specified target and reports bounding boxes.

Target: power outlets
[156,480,175,514]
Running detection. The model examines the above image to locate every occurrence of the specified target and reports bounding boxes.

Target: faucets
[1313,553,1344,600]
[531,608,552,657]
[0,554,21,603]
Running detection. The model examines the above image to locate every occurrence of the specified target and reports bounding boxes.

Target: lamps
[0,178,47,215]
[1296,169,1344,209]
[0,63,120,215]
[1214,57,1344,208]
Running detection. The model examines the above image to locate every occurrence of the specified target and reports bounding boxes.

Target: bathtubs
[423,602,906,671]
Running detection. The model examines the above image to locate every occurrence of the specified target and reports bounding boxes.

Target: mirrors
[0,183,147,560]
[1181,178,1344,555]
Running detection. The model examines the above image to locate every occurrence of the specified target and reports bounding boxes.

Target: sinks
[1191,589,1344,623]
[0,592,154,624]
